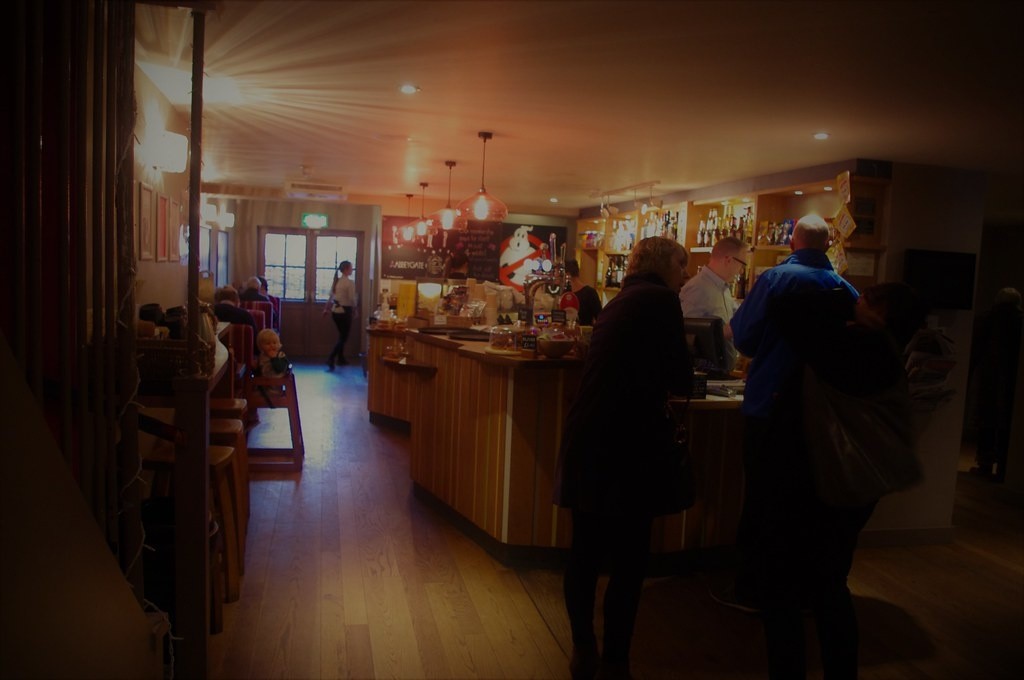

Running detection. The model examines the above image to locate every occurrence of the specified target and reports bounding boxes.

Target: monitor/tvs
[682,314,727,380]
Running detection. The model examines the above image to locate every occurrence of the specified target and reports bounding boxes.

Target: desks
[243,371,304,472]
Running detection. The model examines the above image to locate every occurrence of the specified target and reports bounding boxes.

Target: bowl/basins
[538,338,576,359]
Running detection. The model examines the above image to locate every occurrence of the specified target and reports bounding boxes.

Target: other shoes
[569,646,601,680]
[338,359,350,365]
[597,660,634,680]
[326,360,335,372]
[710,586,763,612]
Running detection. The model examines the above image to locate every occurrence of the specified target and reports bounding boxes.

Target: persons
[967,287,1024,482]
[556,258,602,326]
[212,276,288,397]
[555,236,707,680]
[322,260,359,370]
[730,214,927,680]
[679,236,750,378]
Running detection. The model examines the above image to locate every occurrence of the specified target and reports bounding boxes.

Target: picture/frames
[168,198,181,262]
[155,191,169,262]
[140,182,155,259]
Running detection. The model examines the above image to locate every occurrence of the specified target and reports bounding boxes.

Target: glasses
[725,255,747,270]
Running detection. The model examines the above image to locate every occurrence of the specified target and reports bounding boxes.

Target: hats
[995,286,1022,305]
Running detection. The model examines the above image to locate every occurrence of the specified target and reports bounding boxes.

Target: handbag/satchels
[653,446,695,514]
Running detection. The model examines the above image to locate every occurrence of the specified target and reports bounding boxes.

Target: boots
[989,465,1005,482]
[970,462,993,478]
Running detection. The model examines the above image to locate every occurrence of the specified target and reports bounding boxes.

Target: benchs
[142,289,279,625]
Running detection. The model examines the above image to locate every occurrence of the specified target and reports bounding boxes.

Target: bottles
[639,212,678,244]
[697,205,753,247]
[522,312,536,358]
[382,293,389,319]
[736,266,746,300]
[604,217,637,287]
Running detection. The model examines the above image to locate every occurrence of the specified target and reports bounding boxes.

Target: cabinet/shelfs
[570,159,893,307]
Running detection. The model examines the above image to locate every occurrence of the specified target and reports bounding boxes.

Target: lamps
[600,195,609,218]
[647,185,664,209]
[632,188,648,215]
[606,194,619,215]
[397,131,507,243]
